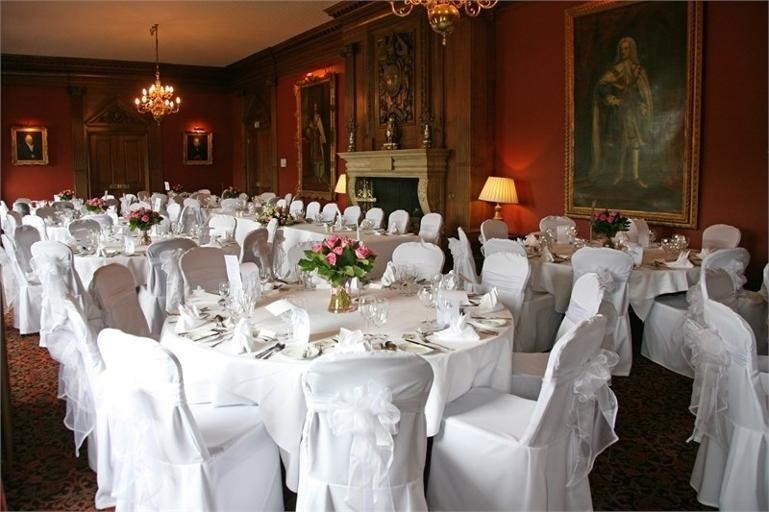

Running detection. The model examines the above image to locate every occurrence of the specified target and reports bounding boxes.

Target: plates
[174,274,507,355]
[321,218,399,236]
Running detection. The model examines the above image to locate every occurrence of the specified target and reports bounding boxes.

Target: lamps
[478,177,519,222]
[334,174,346,194]
[134,24,181,127]
[389,0,499,45]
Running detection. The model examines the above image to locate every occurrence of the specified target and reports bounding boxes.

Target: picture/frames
[182,129,214,166]
[10,126,49,166]
[295,71,337,202]
[564,0,704,230]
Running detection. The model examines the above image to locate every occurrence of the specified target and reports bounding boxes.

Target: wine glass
[219,263,457,340]
[539,226,687,262]
[87,224,216,249]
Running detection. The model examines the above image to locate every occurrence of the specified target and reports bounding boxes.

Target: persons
[20,135,38,159]
[595,37,654,190]
[190,138,201,159]
[307,103,326,183]
